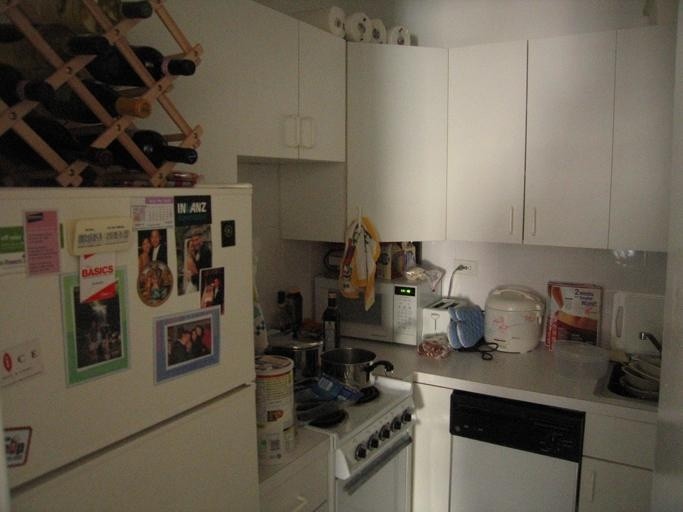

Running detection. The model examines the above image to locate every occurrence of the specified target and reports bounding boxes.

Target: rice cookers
[484,286,545,352]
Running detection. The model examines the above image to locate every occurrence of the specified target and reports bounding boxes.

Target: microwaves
[312,270,442,347]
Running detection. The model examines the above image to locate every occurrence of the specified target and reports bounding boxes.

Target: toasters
[422,296,466,338]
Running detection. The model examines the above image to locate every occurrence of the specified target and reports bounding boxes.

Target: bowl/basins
[619,359,661,399]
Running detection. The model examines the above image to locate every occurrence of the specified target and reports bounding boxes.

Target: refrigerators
[0,183,260,512]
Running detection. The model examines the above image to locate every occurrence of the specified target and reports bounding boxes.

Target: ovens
[447,390,585,512]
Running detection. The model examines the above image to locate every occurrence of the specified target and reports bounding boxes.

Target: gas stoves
[294,384,413,481]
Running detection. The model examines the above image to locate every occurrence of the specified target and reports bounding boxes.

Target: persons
[184,231,210,290]
[203,277,222,310]
[83,312,121,367]
[148,230,166,264]
[170,323,210,363]
[200,282,214,308]
[137,237,151,272]
[182,239,197,294]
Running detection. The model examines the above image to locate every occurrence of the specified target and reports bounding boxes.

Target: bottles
[272,285,302,334]
[1,0,198,169]
[320,289,343,353]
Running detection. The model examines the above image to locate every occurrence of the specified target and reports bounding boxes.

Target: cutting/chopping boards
[610,289,665,358]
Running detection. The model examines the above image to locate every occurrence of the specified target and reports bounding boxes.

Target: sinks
[600,357,659,407]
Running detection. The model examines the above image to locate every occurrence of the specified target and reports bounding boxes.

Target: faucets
[638,330,662,353]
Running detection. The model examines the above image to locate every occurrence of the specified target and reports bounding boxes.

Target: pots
[265,329,393,390]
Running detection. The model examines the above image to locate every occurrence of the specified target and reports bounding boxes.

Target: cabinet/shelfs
[237,0,346,165]
[576,399,659,512]
[447,30,617,251]
[609,22,677,253]
[280,42,449,242]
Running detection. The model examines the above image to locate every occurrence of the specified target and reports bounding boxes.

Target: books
[542,280,602,351]
